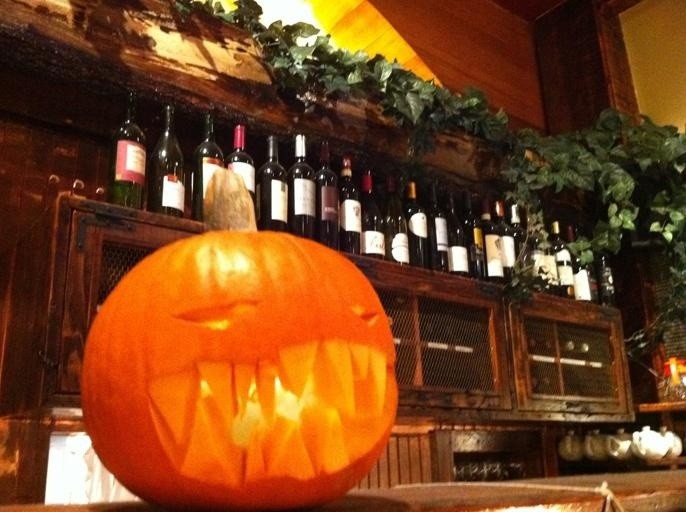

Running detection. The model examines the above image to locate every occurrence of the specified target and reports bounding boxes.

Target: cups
[454,462,528,480]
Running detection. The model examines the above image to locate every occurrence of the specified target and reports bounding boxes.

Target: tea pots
[557,426,682,461]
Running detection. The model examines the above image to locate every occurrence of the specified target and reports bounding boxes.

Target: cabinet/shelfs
[0,2,686,512]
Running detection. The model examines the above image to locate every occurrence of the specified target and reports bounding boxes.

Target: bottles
[338,155,362,255]
[424,182,449,271]
[110,92,148,210]
[445,190,469,277]
[225,124,256,216]
[255,134,288,232]
[316,139,339,251]
[359,168,386,261]
[287,134,317,236]
[147,103,187,217]
[189,109,224,222]
[403,181,428,266]
[460,192,615,306]
[381,176,411,264]
